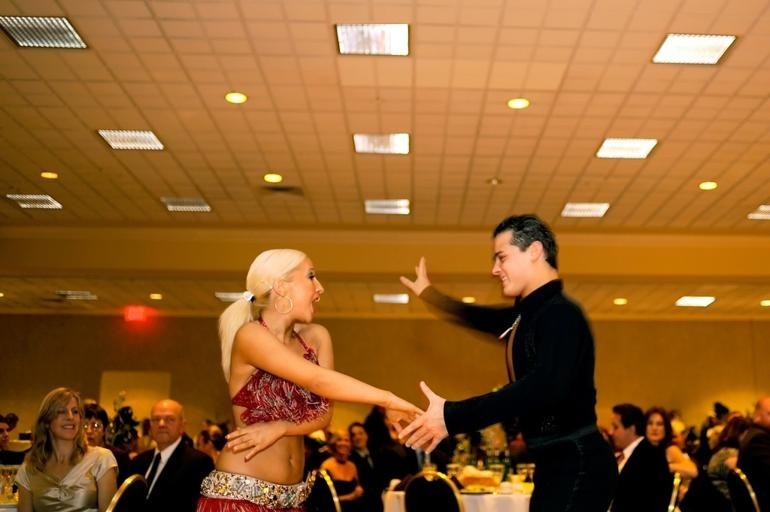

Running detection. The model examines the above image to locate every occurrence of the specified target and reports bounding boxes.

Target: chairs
[667,469,763,511]
[99,473,151,512]
[305,465,467,512]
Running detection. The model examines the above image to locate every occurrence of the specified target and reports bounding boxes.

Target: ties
[145,453,160,497]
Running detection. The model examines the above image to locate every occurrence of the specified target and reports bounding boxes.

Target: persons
[610,397,770,512]
[193,248,432,511]
[398,214,619,511]
[0,388,231,512]
[303,405,530,511]
